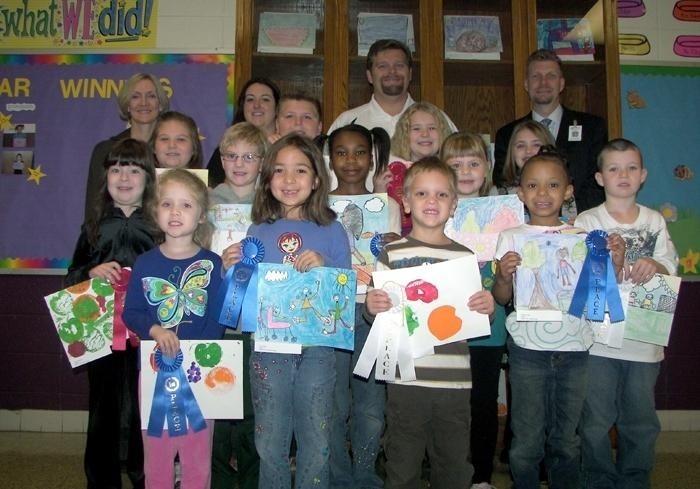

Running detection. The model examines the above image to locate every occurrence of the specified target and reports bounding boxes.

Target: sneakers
[470,481,499,489]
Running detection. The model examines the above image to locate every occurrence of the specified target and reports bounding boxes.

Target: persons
[205,119,265,488]
[307,122,404,489]
[319,38,459,192]
[495,117,578,232]
[493,47,608,219]
[367,102,456,243]
[567,137,681,487]
[84,71,172,225]
[63,137,159,489]
[9,151,26,174]
[203,77,282,191]
[492,144,629,487]
[12,123,29,146]
[263,94,329,167]
[436,127,509,489]
[119,166,230,489]
[217,131,351,489]
[150,111,205,173]
[361,157,497,489]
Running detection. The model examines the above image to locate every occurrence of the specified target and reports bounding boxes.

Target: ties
[540,118,553,130]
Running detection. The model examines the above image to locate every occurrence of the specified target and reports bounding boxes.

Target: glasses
[220,151,263,162]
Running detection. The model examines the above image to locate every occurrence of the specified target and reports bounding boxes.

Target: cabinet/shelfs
[233,0,623,144]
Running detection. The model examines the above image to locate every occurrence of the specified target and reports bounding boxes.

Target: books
[357,11,417,56]
[257,11,316,55]
[536,18,596,61]
[443,14,504,61]
